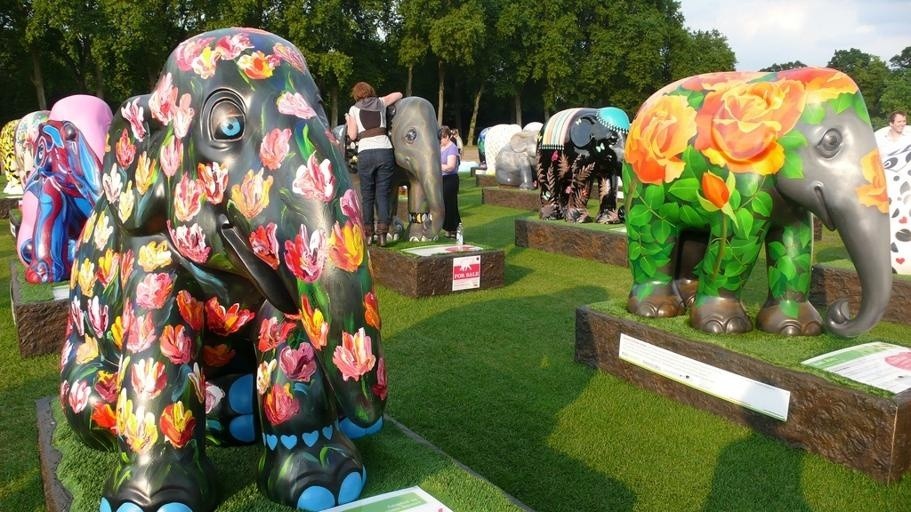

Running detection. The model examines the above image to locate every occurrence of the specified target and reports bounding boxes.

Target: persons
[345,82,404,247]
[877,111,909,158]
[441,126,463,237]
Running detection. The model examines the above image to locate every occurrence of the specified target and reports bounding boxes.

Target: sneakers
[442,230,457,240]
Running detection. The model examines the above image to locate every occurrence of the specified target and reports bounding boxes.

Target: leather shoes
[363,232,388,248]
